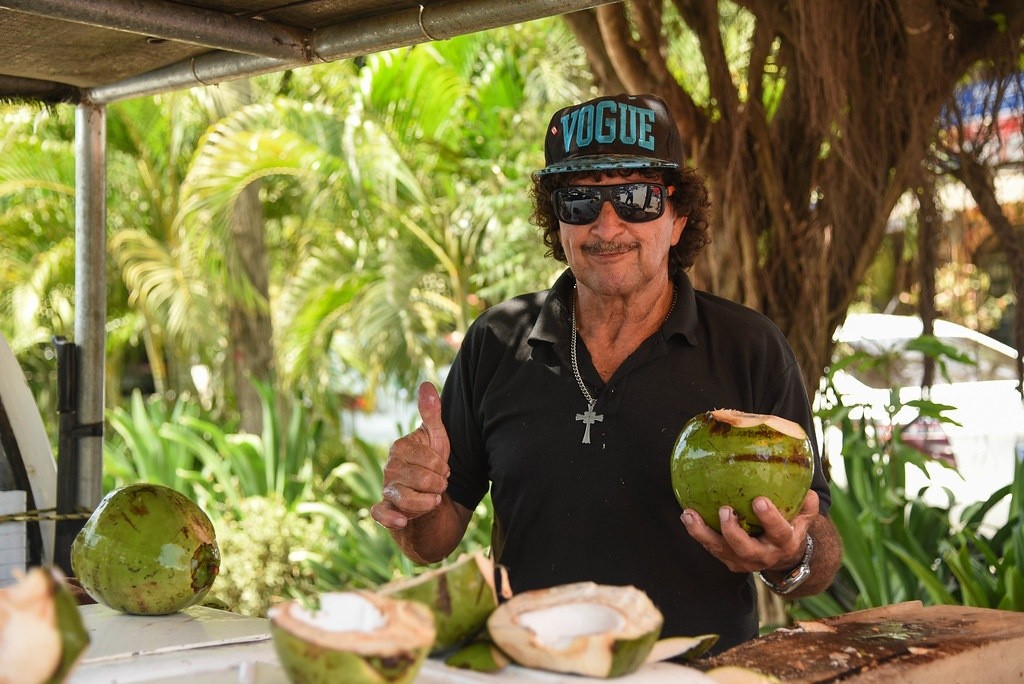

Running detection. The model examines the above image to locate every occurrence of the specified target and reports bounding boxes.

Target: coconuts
[265,548,663,684]
[671,408,814,538]
[68,482,221,615]
[0,563,92,684]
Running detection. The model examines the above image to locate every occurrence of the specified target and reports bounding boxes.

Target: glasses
[551,182,676,225]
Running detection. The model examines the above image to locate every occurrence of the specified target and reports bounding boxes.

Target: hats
[536,93,682,175]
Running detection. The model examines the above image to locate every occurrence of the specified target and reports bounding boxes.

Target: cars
[810,313,1024,538]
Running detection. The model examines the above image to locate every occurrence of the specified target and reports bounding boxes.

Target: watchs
[758,535,813,595]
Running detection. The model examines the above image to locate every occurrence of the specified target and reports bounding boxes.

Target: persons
[372,95,842,659]
[625,187,633,203]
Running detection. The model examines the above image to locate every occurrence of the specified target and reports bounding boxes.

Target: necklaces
[569,282,678,446]
